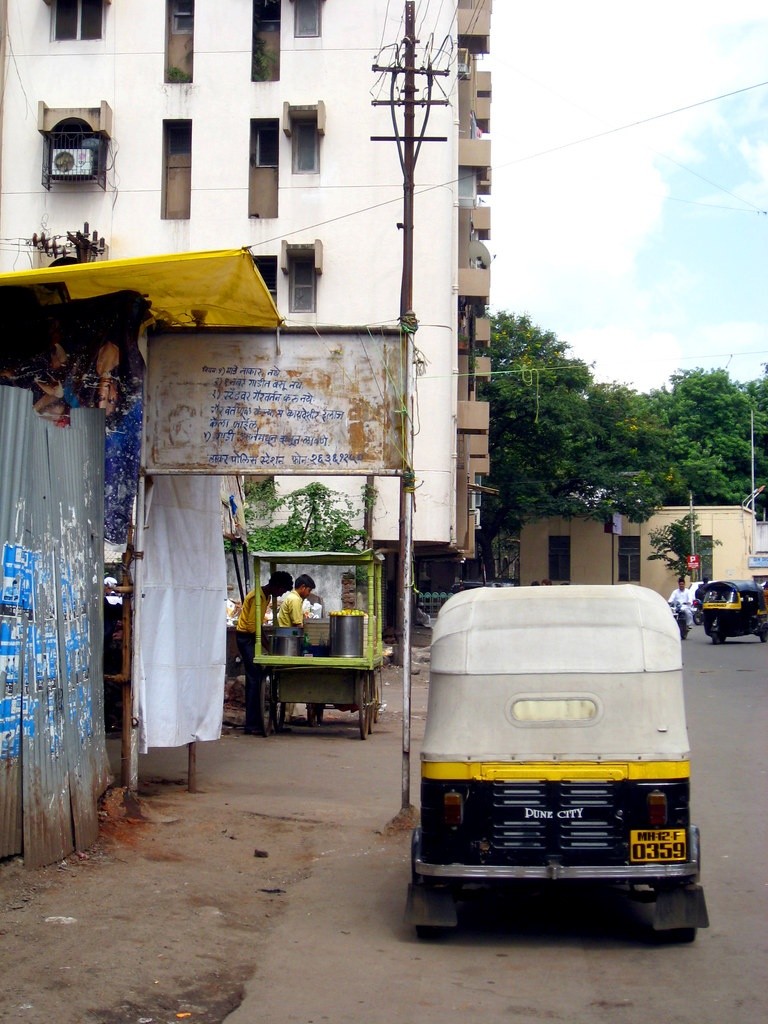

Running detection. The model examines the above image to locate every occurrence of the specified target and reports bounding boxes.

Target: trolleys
[247,546,390,743]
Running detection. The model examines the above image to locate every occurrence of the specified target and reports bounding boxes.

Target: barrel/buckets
[304,613,377,661]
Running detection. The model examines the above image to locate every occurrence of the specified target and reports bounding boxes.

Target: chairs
[418,592,453,613]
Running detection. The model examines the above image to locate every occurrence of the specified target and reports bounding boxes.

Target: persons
[103,576,133,642]
[668,576,694,629]
[697,577,709,602]
[233,567,296,736]
[532,579,569,587]
[275,573,318,628]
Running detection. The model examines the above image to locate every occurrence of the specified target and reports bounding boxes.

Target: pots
[269,635,305,657]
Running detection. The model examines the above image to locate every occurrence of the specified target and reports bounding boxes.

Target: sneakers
[686,625,692,631]
[245,726,276,736]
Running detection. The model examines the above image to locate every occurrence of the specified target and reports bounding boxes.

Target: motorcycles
[700,579,768,648]
[666,600,692,640]
[401,582,712,946]
[691,596,707,626]
